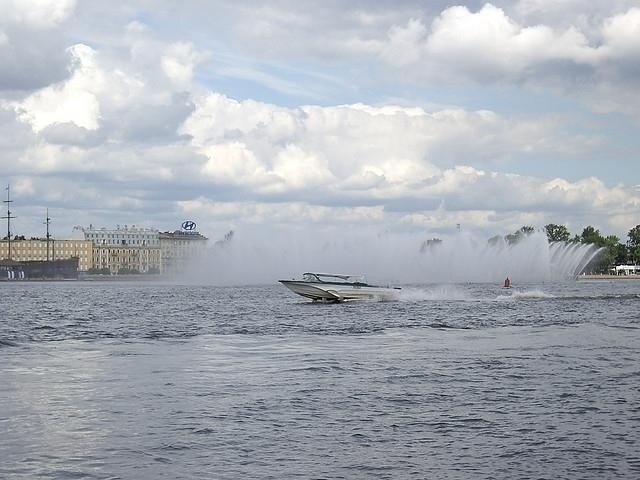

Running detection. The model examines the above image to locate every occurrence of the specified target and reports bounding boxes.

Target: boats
[277,272,403,302]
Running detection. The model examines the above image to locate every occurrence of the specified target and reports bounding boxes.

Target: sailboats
[0,183,80,281]
